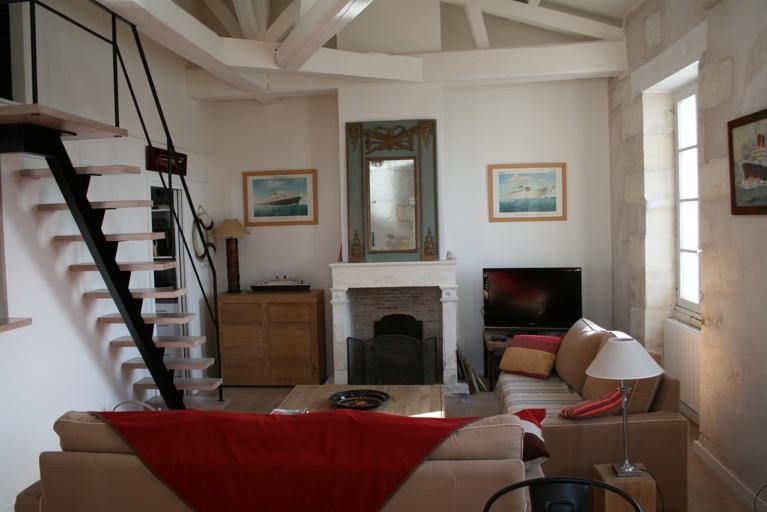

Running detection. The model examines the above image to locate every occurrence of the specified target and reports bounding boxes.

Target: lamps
[583,337,666,476]
[213,218,251,294]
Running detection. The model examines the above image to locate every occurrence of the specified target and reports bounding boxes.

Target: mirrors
[345,119,440,261]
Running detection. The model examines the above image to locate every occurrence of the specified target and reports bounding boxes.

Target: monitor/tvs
[483,267,582,333]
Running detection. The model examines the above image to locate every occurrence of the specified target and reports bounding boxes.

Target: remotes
[499,335,506,342]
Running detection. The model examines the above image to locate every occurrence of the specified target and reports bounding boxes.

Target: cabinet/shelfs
[216,290,326,385]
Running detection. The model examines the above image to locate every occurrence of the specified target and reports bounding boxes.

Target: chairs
[482,476,645,512]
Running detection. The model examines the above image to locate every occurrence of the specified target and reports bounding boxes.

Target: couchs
[492,372,688,512]
[15,410,547,511]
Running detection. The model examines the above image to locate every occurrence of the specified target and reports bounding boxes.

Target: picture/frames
[242,170,320,227]
[486,163,568,222]
[727,109,767,215]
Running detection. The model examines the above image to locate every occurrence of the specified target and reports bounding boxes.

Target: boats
[499,185,549,201]
[740,132,766,183]
[255,192,303,208]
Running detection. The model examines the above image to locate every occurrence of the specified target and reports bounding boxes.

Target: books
[456,346,488,393]
[271,407,309,415]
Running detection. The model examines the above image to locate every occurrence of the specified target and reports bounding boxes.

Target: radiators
[663,315,707,425]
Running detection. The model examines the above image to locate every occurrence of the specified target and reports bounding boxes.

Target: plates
[328,388,390,410]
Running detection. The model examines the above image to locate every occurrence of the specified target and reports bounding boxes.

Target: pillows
[497,316,662,420]
